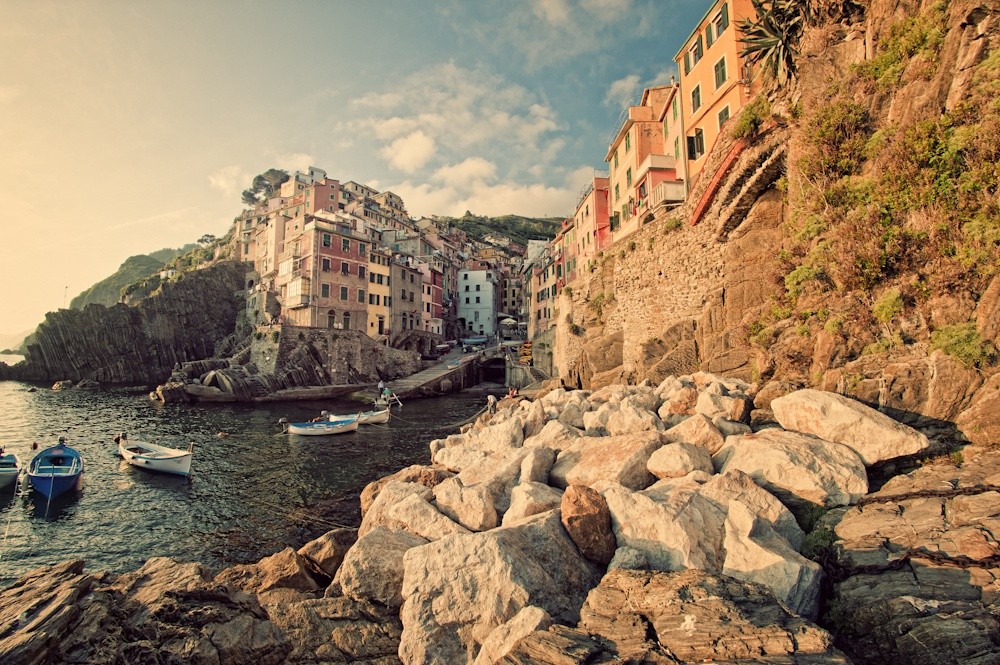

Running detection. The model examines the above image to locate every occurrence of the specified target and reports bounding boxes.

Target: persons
[378,381,384,397]
[383,388,391,404]
[486,393,497,414]
[500,387,518,399]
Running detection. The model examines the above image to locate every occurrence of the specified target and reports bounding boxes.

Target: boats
[26,435,84,501]
[118,431,195,477]
[0,445,23,490]
[321,403,392,425]
[279,411,362,436]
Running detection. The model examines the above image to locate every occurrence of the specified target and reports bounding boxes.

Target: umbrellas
[500,318,517,329]
